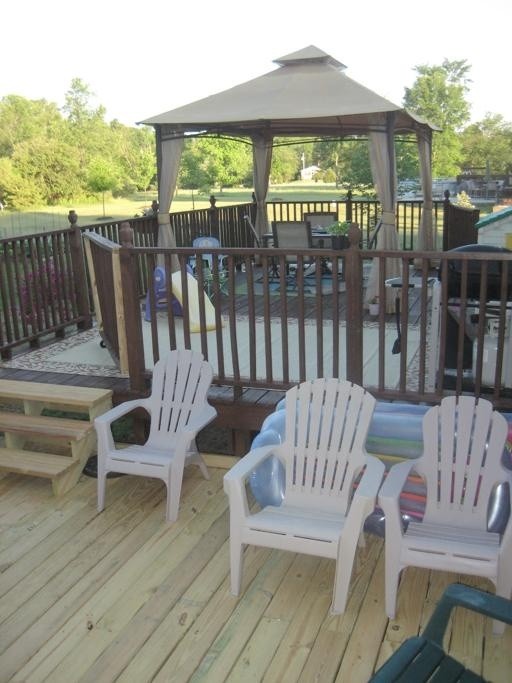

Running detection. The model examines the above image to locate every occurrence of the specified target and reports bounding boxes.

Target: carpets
[229,273,349,297]
[1,313,432,393]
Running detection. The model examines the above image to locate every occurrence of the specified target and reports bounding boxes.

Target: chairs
[243,212,382,283]
